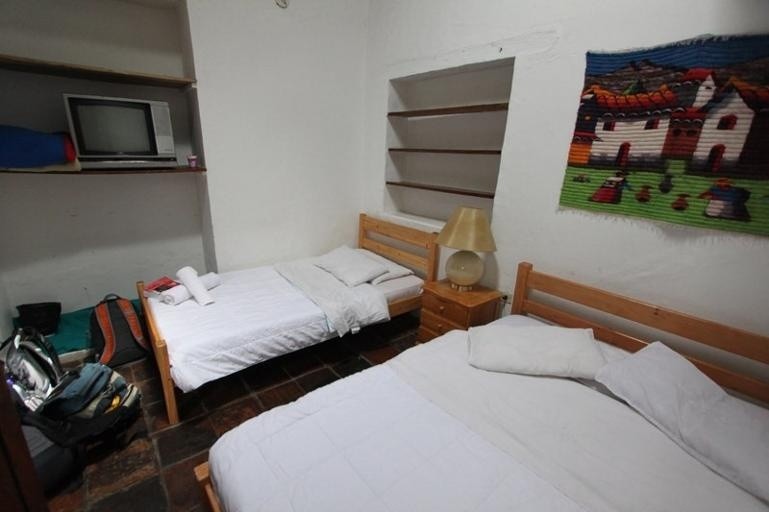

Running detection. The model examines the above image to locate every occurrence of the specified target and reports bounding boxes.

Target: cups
[187,156,197,168]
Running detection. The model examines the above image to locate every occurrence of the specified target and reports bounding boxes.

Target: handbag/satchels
[0,325,144,500]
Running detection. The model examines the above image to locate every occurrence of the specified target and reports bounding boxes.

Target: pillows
[313,244,416,288]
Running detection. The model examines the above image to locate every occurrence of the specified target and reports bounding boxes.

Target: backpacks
[89,293,155,368]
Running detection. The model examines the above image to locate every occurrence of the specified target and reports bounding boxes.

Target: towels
[158,266,220,307]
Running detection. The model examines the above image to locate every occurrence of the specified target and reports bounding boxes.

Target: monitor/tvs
[61,93,178,168]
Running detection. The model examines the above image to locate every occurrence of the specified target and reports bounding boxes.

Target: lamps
[433,206,496,296]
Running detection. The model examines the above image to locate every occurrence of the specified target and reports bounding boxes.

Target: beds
[195,261,768,512]
[136,210,439,426]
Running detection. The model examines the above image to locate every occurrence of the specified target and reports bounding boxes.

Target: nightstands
[418,278,508,342]
